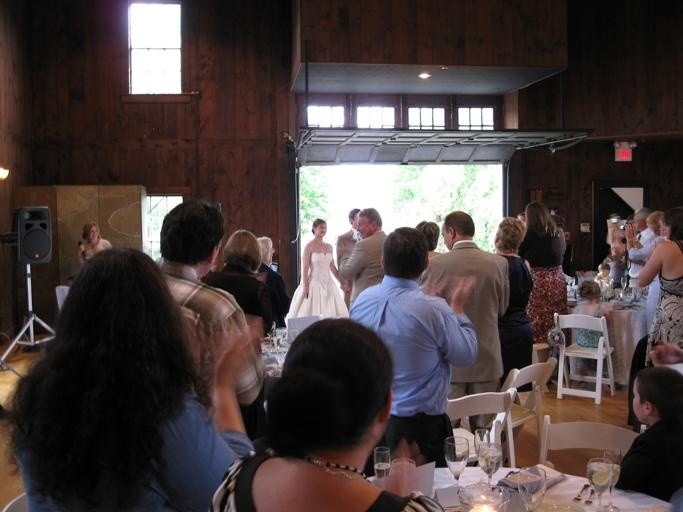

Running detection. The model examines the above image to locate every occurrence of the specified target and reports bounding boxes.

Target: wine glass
[602,445,624,511]
[263,322,287,374]
[620,276,627,296]
[587,456,612,511]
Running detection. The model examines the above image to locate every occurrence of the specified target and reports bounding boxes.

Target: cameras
[619,222,632,231]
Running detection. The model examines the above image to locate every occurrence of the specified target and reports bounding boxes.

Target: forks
[574,484,589,500]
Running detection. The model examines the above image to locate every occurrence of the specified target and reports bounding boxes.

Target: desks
[567,289,646,387]
[365,466,672,512]
[259,329,292,396]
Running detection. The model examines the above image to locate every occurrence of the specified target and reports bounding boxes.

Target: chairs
[577,271,596,284]
[2,490,28,512]
[448,313,648,477]
[287,316,319,341]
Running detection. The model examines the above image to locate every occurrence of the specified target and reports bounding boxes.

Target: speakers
[12,205,54,266]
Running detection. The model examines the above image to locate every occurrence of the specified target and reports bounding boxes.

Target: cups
[373,427,549,511]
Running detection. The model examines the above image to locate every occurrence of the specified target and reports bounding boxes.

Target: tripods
[0,269,57,367]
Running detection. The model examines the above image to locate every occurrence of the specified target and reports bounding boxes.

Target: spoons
[585,488,594,503]
